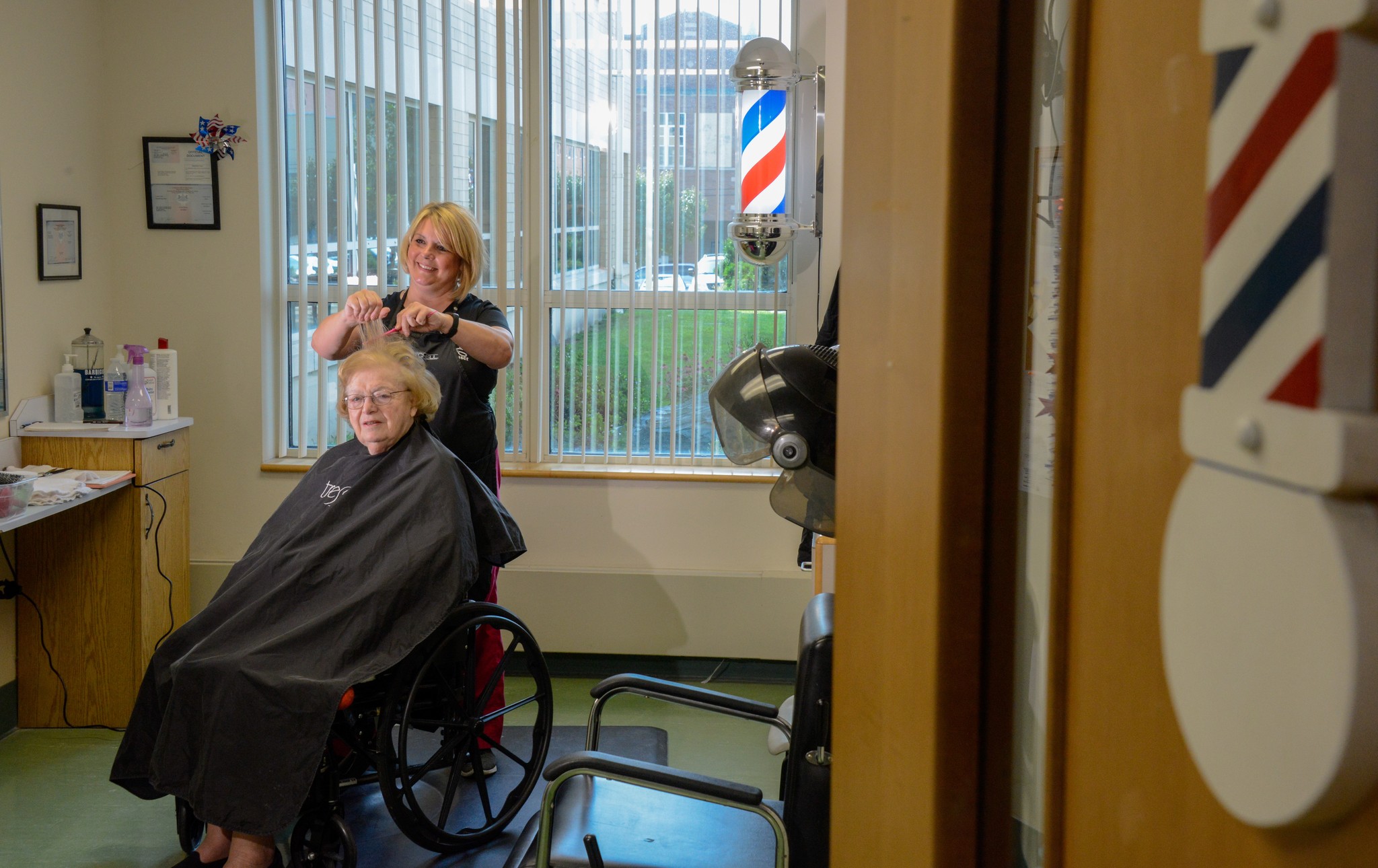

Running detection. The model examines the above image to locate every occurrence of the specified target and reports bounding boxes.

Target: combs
[362,310,438,343]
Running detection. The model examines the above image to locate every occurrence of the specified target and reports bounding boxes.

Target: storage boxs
[0,471,38,523]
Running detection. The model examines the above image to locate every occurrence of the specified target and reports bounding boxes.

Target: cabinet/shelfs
[18,417,194,731]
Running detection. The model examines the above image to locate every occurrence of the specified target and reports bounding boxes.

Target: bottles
[128,362,159,421]
[149,338,178,420]
[104,357,128,423]
[71,328,105,421]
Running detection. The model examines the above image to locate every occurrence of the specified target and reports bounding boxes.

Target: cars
[633,253,728,292]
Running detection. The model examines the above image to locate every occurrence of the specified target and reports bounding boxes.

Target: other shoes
[458,748,497,777]
[171,850,229,868]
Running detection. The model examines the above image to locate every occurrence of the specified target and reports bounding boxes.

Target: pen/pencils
[72,420,124,424]
[20,421,43,428]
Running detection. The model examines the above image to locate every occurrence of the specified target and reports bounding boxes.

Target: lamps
[727,38,824,265]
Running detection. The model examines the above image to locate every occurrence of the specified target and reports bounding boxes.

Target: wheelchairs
[173,563,556,868]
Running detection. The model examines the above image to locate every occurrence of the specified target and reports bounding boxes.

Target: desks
[0,468,132,534]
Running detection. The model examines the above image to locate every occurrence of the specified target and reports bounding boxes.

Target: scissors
[37,466,74,478]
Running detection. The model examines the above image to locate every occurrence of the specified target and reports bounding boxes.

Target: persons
[114,301,526,868]
[310,201,519,776]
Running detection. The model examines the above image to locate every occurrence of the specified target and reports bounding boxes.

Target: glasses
[342,389,411,409]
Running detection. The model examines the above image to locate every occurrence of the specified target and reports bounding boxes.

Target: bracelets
[440,312,459,338]
